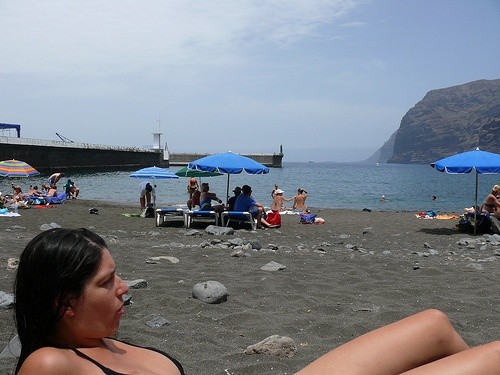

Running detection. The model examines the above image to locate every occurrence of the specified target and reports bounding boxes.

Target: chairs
[156,208,259,231]
[23,192,66,204]
[463,211,500,230]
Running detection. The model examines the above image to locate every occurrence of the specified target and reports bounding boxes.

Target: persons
[481,184,500,217]
[0,172,80,209]
[13,227,500,375]
[271,190,296,212]
[187,176,222,219]
[381,195,386,202]
[271,185,280,198]
[138,182,157,210]
[432,195,438,201]
[227,185,267,229]
[291,189,309,211]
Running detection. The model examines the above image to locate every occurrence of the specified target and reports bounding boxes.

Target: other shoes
[258,225,265,229]
[269,224,280,228]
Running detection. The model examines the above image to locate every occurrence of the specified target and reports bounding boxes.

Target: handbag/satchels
[300,214,317,224]
[266,210,281,225]
[143,207,156,218]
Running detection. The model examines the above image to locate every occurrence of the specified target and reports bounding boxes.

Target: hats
[275,189,284,194]
[233,187,241,193]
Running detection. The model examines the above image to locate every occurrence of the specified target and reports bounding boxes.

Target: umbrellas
[429,147,500,235]
[0,159,41,192]
[175,166,224,187]
[187,150,269,207]
[128,165,179,179]
